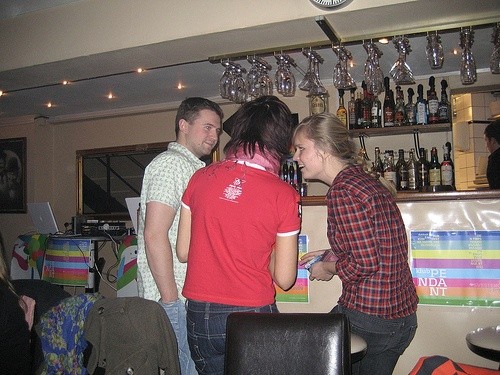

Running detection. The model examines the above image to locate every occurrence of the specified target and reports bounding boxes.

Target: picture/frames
[0,136,28,214]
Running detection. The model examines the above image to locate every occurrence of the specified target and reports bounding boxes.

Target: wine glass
[490,23,500,75]
[389,37,416,85]
[425,35,444,68]
[220,43,384,104]
[458,28,476,85]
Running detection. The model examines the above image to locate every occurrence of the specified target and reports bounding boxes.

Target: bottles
[439,88,451,123]
[383,150,395,186]
[416,148,429,193]
[372,92,382,127]
[371,147,383,179]
[306,82,330,115]
[394,92,404,126]
[428,146,453,192]
[406,152,416,192]
[355,91,362,129]
[416,92,427,125]
[383,91,394,128]
[348,93,356,130]
[337,97,347,130]
[404,95,416,126]
[362,90,372,129]
[395,149,408,192]
[427,86,439,124]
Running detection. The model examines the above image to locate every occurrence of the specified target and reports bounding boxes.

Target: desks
[465,327,500,370]
[15,230,126,293]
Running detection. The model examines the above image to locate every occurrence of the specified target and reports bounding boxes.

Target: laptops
[26,201,82,237]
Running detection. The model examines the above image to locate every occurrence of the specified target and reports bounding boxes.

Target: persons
[0,239,44,375]
[178,95,301,375]
[484,119,500,190]
[292,112,419,375]
[137,97,223,375]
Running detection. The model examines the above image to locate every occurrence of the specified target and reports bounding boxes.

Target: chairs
[224,312,352,375]
